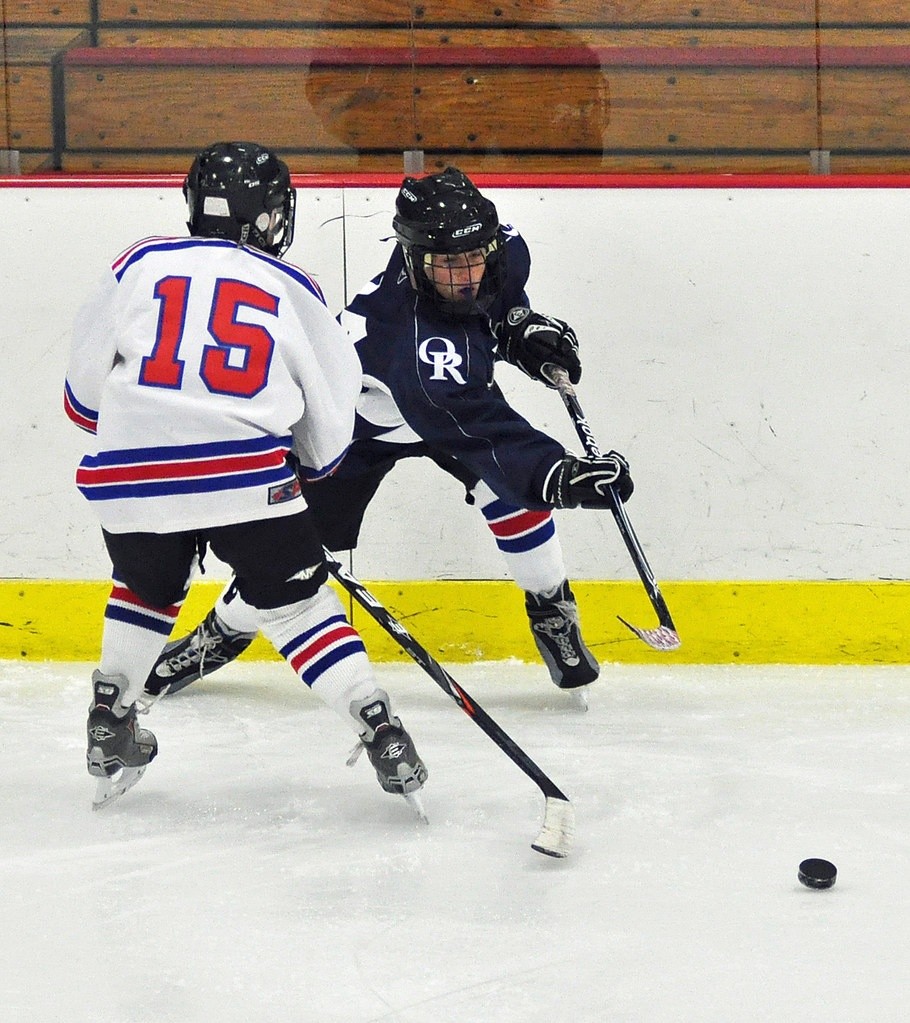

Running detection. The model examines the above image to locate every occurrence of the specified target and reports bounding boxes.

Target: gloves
[543,451,634,509]
[498,306,582,390]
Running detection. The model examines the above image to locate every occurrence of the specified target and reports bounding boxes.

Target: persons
[143,166,636,710]
[62,138,431,826]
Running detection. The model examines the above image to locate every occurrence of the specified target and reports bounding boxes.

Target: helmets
[182,141,296,260]
[392,167,508,317]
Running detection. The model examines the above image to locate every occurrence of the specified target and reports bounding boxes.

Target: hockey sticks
[318,542,577,860]
[545,364,683,653]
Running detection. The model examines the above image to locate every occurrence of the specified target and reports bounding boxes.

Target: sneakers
[526,577,600,712]
[142,607,258,701]
[86,669,171,812]
[345,688,430,826]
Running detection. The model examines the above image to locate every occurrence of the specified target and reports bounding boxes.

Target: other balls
[797,857,838,890]
